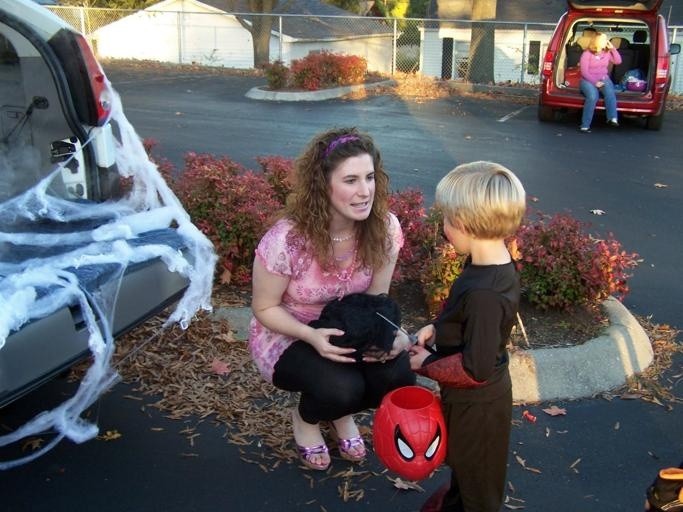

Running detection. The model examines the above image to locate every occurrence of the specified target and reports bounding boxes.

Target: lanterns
[376,312,444,480]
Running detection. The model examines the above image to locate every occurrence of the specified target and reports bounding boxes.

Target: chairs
[567,28,649,83]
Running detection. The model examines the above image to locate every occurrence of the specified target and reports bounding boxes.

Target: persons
[249,126,416,471]
[404,161,525,512]
[578,31,622,133]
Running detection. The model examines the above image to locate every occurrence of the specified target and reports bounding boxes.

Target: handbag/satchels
[566,43,583,67]
[647,468,683,512]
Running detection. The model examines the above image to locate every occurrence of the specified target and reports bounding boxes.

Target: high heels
[293,420,366,470]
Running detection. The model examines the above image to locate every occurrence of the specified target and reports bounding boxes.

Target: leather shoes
[607,120,618,126]
[576,127,592,132]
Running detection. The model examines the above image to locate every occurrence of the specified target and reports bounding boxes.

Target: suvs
[535,0,671,130]
[0,0,215,411]
